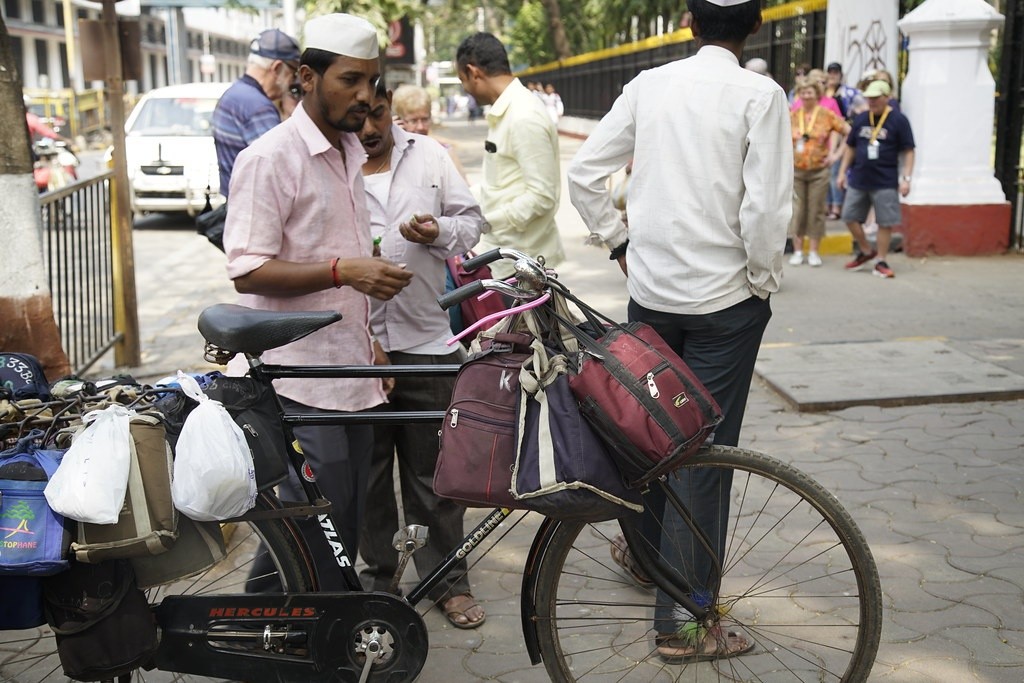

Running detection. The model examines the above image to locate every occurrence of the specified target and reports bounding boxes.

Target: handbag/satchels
[0,352,290,683]
[195,199,230,254]
[432,251,722,524]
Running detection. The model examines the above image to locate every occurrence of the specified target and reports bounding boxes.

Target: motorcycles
[30,118,80,220]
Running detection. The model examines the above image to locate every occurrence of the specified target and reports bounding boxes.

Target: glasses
[271,59,300,78]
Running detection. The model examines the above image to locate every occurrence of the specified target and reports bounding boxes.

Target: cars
[103,82,233,222]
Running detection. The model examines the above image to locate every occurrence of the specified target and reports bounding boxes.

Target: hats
[828,63,841,72]
[862,80,891,98]
[249,29,302,60]
[303,11,379,60]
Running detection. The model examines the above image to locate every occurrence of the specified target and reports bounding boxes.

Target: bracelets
[371,335,378,342]
[331,258,342,289]
[903,176,911,182]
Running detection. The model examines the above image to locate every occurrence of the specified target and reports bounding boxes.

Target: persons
[748,58,916,277]
[393,83,468,183]
[569,0,794,665]
[210,25,301,199]
[358,75,486,627]
[526,81,564,123]
[21,94,64,141]
[456,31,566,278]
[223,13,413,592]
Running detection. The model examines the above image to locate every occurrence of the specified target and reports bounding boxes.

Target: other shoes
[807,251,822,267]
[824,209,841,220]
[790,250,803,266]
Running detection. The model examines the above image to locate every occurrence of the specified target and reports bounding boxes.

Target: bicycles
[0,242,884,683]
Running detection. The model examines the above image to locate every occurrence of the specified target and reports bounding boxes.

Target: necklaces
[376,134,393,173]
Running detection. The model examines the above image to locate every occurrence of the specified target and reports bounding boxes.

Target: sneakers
[845,251,878,271]
[872,261,894,278]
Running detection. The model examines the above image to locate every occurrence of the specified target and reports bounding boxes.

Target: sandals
[440,594,486,629]
[611,531,657,586]
[655,625,754,664]
